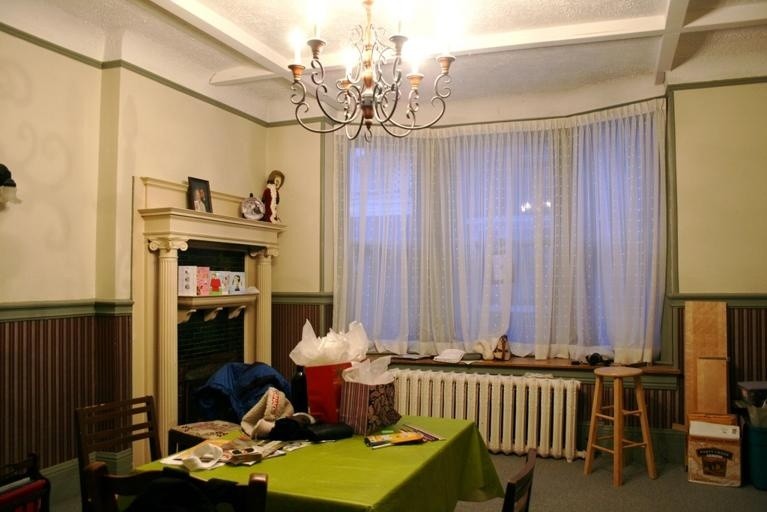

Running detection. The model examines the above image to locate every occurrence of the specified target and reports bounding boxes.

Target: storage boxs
[670,418,745,488]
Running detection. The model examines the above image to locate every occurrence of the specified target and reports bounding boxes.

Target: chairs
[70,395,268,512]
[454,446,538,512]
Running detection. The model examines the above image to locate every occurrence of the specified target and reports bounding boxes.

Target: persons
[194,187,209,213]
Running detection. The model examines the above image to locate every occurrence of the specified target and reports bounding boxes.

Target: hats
[240,386,295,441]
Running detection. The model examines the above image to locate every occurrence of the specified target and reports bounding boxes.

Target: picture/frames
[187,176,213,213]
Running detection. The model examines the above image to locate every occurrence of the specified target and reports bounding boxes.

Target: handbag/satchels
[303,361,402,442]
[492,334,512,361]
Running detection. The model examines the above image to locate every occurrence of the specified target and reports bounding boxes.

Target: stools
[586,366,659,488]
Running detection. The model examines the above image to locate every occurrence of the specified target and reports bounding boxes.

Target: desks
[133,412,479,512]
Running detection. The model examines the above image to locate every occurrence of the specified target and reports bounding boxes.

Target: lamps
[287,0,456,141]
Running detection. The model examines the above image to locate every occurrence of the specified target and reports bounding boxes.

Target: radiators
[387,368,586,463]
